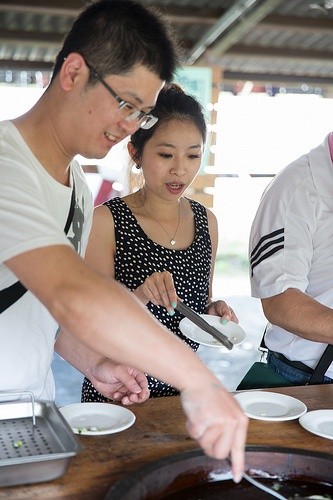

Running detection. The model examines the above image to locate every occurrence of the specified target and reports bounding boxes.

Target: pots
[97,444,333,500]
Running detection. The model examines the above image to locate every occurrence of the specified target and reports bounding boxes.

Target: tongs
[173,298,233,351]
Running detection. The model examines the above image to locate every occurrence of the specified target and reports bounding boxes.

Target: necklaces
[137,189,180,246]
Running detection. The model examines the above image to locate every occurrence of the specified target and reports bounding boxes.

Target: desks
[0,383,333,500]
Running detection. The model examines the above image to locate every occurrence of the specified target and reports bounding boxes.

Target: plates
[58,402,136,436]
[180,313,245,347]
[299,409,333,441]
[233,391,307,422]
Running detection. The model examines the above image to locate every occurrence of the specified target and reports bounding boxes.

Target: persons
[0,0,249,483]
[81,82,239,403]
[250,132,333,387]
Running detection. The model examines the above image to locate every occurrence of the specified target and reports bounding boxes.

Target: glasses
[85,64,158,130]
